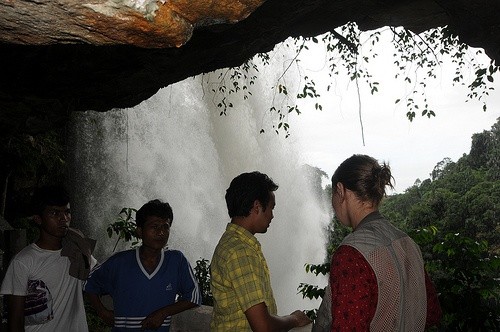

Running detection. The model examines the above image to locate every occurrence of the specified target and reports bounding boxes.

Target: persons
[209,172,313,332]
[311,155,443,332]
[0,183,101,332]
[84,198,202,332]
